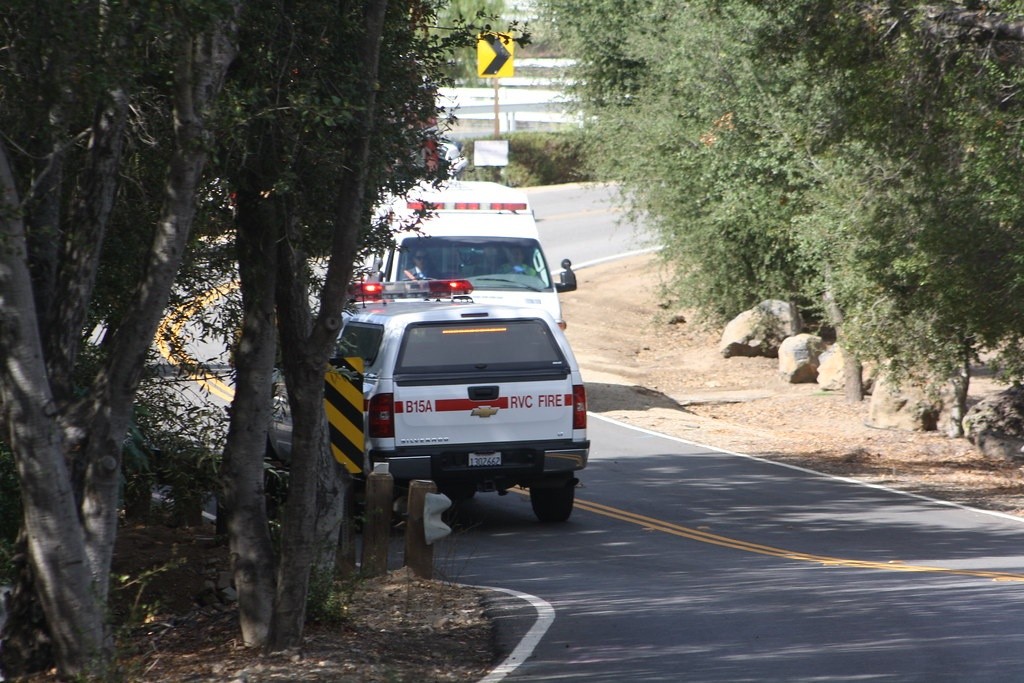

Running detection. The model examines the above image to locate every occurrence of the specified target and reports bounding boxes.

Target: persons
[400,249,439,280]
[497,246,536,277]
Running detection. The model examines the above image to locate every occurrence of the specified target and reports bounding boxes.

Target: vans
[265,279,592,525]
[353,175,580,336]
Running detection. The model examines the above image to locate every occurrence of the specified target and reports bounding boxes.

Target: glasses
[414,255,429,261]
